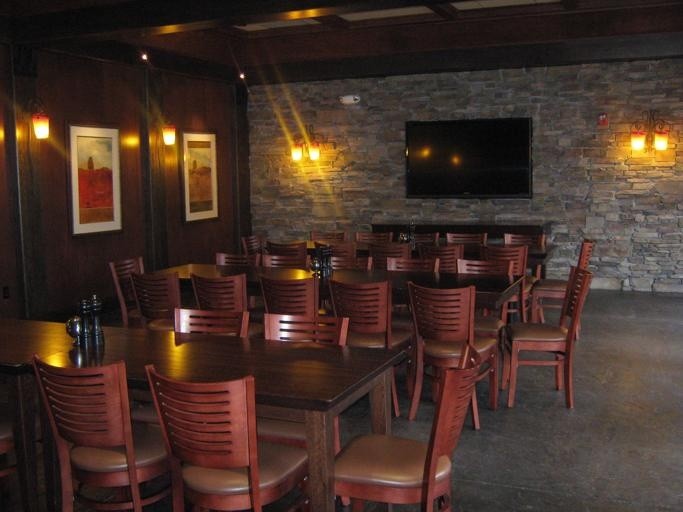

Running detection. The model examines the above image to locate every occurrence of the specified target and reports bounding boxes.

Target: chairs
[251,313,352,507]
[307,342,485,512]
[32,350,172,512]
[137,361,313,511]
[106,220,596,428]
[0,360,47,512]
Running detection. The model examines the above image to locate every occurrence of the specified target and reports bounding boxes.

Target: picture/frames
[177,129,222,227]
[63,119,127,238]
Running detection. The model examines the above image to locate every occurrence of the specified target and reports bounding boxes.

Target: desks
[0,314,408,512]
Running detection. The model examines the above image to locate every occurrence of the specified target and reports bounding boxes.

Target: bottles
[91,335,103,364]
[76,336,88,367]
[77,299,90,337]
[319,258,330,271]
[88,294,102,336]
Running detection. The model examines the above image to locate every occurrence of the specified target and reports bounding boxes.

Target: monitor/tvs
[404,115,533,199]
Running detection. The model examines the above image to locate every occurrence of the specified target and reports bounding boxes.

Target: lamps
[22,94,53,141]
[151,110,179,147]
[628,108,671,158]
[289,121,338,162]
